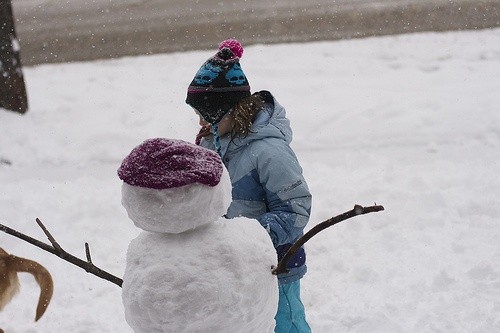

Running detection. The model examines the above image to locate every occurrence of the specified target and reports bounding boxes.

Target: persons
[186,38,310,332]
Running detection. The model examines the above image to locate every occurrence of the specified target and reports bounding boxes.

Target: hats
[185,38,251,125]
